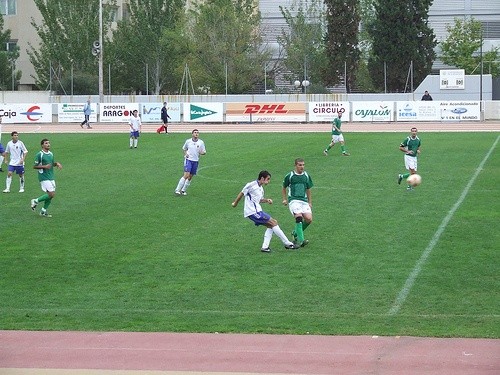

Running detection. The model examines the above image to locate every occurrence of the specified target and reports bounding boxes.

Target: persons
[128,109,142,149]
[421,90,433,101]
[2,132,29,193]
[29,138,63,219]
[80,96,93,130]
[156,101,172,134]
[231,169,301,253]
[397,127,422,192]
[0,142,6,172]
[172,128,207,197]
[322,111,351,157]
[280,157,315,249]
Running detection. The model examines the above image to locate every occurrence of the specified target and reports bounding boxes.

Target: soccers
[406,174,422,186]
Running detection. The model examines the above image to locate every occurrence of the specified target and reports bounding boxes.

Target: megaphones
[91,47,101,56]
[93,40,101,48]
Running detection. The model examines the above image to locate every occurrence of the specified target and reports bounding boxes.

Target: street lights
[8,59,17,91]
[70,59,75,95]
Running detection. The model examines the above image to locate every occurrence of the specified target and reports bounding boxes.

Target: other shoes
[342,152,350,156]
[157,130,161,134]
[398,173,403,185]
[87,127,92,129]
[165,132,169,134]
[2,189,11,193]
[130,146,132,149]
[323,150,328,156]
[175,189,180,195]
[301,240,309,247]
[407,186,415,191]
[291,230,298,241]
[80,123,83,128]
[261,248,271,252]
[39,210,53,218]
[285,244,298,249]
[31,199,37,212]
[181,190,188,196]
[19,186,25,193]
[0,168,4,172]
[134,146,138,149]
